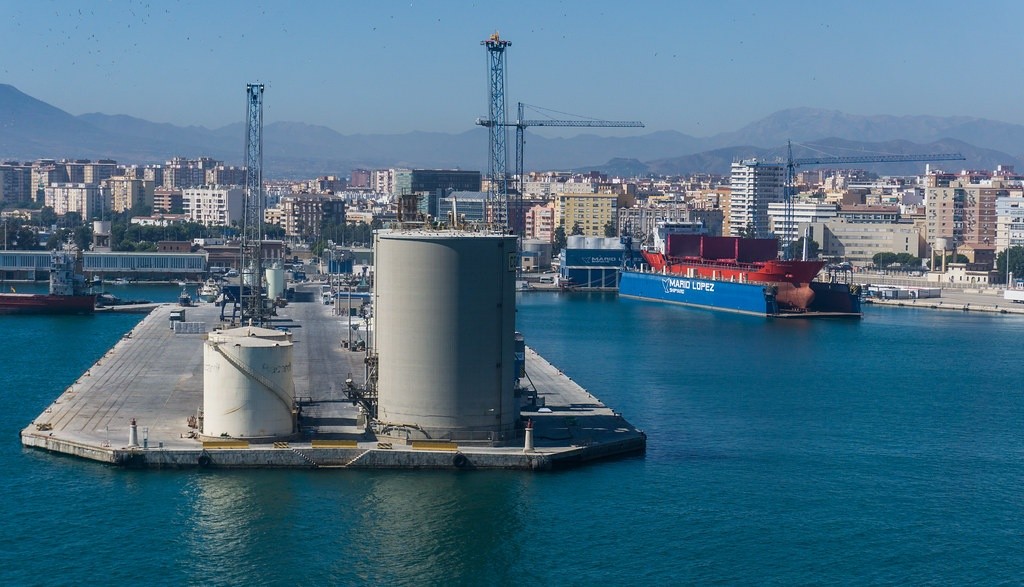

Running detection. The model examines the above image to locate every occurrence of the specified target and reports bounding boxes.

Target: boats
[616,221,865,320]
[0,247,97,317]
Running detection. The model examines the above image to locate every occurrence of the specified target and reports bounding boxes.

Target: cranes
[737,137,968,264]
[474,101,646,282]
